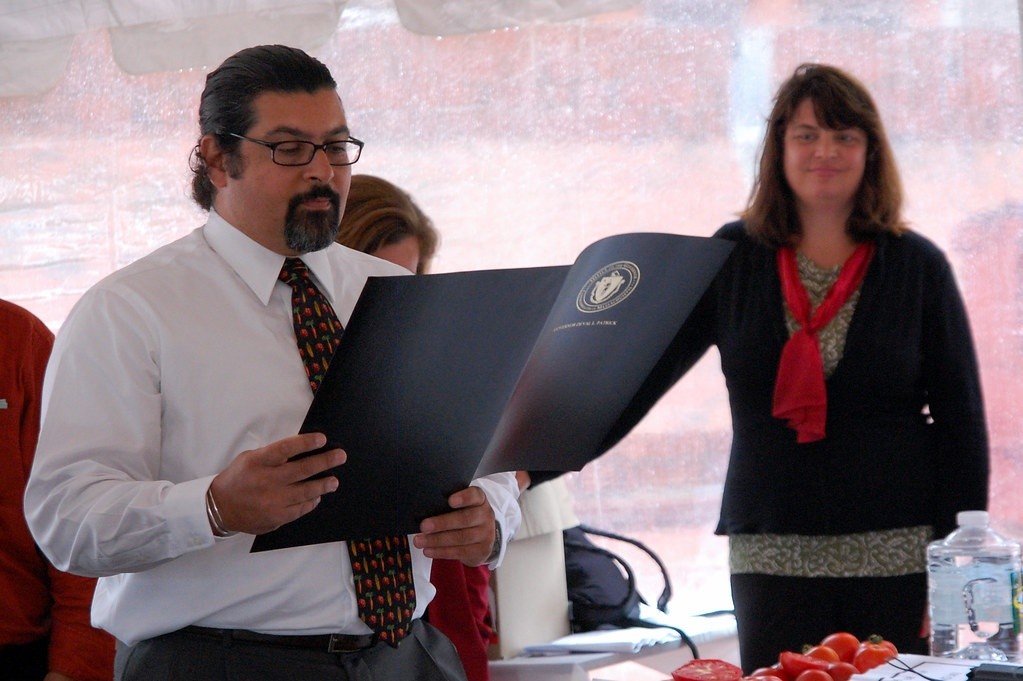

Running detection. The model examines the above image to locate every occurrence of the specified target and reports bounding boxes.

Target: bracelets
[206,484,234,537]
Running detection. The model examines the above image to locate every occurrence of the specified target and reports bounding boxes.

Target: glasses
[228,131,365,168]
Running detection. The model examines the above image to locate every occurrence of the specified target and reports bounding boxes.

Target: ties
[276,255,418,650]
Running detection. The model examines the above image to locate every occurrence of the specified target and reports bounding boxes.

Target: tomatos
[670,633,899,681]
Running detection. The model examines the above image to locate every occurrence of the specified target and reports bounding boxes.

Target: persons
[22,45,524,680]
[338,173,499,681]
[1,297,117,681]
[516,59,991,675]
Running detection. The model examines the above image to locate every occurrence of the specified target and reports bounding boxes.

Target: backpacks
[557,522,672,628]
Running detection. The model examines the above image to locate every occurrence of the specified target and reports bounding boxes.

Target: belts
[175,622,384,655]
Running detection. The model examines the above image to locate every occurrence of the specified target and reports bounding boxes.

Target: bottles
[926,510,1023,667]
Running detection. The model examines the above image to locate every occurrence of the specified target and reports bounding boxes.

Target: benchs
[488,614,737,681]
